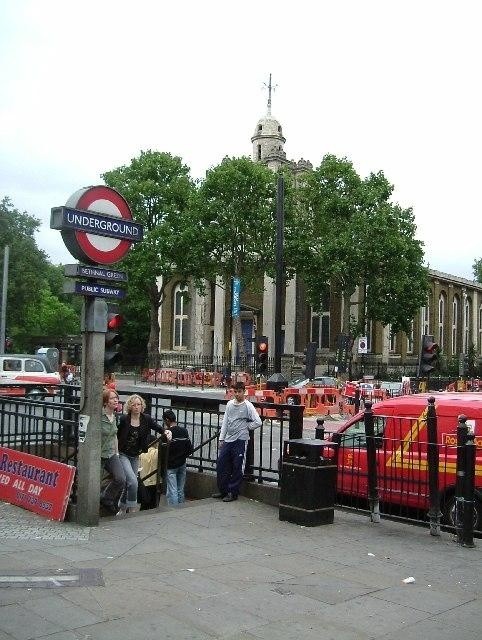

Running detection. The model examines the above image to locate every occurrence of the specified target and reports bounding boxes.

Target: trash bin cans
[279,438,337,527]
[266,373,288,403]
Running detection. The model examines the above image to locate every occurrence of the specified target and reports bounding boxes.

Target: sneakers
[212,491,238,501]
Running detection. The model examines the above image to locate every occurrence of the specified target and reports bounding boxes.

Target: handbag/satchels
[164,438,191,459]
[138,477,150,505]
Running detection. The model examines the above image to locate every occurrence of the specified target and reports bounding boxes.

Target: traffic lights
[417,335,439,378]
[104,302,125,373]
[302,343,316,379]
[255,336,268,373]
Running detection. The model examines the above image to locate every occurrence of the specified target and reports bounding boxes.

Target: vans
[277,393,482,536]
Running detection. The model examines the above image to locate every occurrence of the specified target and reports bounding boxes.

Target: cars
[286,377,404,405]
[1,354,62,404]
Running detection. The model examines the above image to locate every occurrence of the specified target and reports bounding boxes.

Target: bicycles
[338,396,355,419]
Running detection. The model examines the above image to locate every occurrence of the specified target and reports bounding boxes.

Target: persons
[138,434,164,511]
[158,409,193,505]
[61,361,70,384]
[67,363,76,378]
[116,393,165,515]
[100,388,127,515]
[211,381,263,502]
[52,455,58,461]
